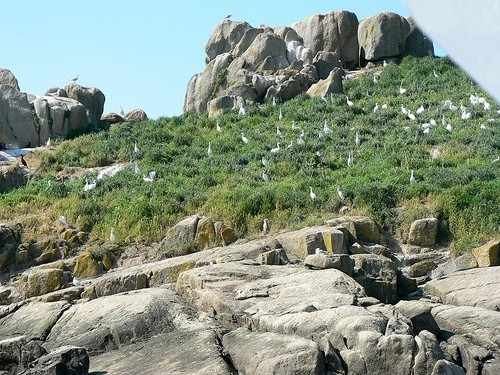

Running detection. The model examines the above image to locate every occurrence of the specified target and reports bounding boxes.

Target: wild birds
[315,247,329,255]
[109,227,117,245]
[309,186,317,205]
[134,140,156,185]
[59,214,67,225]
[72,276,85,287]
[0,137,7,151]
[224,13,233,21]
[20,154,28,167]
[337,184,347,201]
[46,137,52,151]
[82,178,97,198]
[260,218,268,235]
[68,74,81,84]
[118,107,125,116]
[206,60,500,184]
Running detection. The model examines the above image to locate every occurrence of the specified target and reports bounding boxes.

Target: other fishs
[25,139,32,149]
